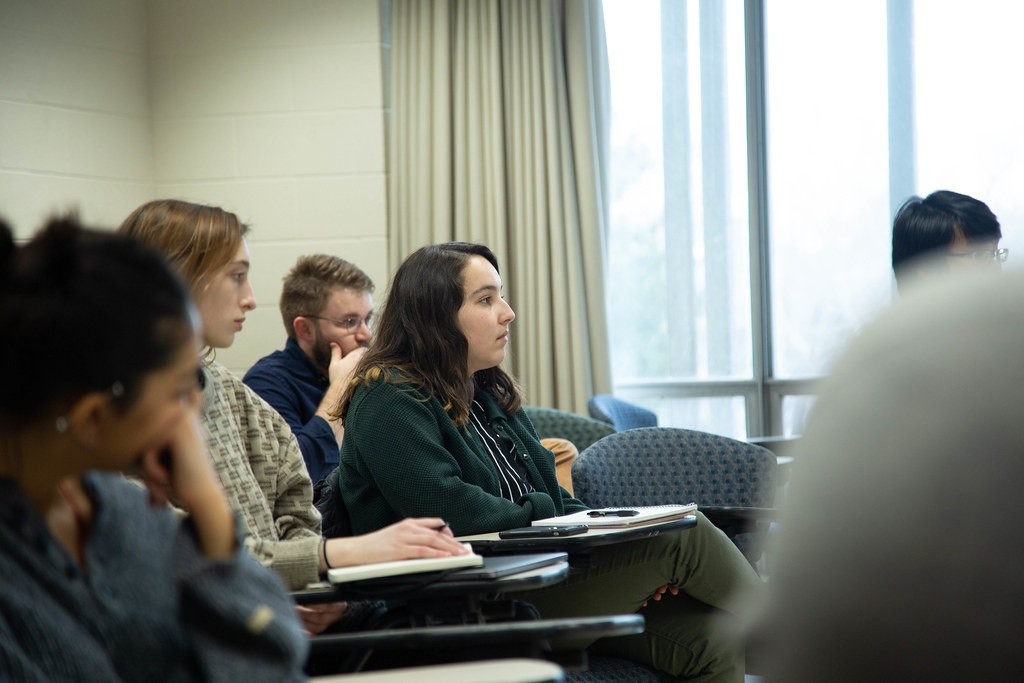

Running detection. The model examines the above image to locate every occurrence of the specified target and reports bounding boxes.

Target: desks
[292,515,697,671]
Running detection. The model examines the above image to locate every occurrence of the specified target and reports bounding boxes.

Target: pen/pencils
[587,510,640,518]
[433,523,449,532]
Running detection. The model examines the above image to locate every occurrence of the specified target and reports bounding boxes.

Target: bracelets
[322,537,334,568]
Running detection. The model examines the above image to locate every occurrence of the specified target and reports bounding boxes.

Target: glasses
[300,315,374,333]
[950,248,1009,264]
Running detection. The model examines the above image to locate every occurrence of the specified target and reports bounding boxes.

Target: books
[328,542,482,583]
[533,506,698,530]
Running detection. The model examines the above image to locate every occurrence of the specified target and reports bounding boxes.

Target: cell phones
[499,524,589,539]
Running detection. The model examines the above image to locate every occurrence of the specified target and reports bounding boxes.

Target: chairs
[524,395,780,509]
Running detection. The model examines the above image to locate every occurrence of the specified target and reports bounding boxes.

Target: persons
[715,191,1024,683]
[1,219,307,683]
[327,243,765,683]
[242,251,377,484]
[117,200,468,680]
